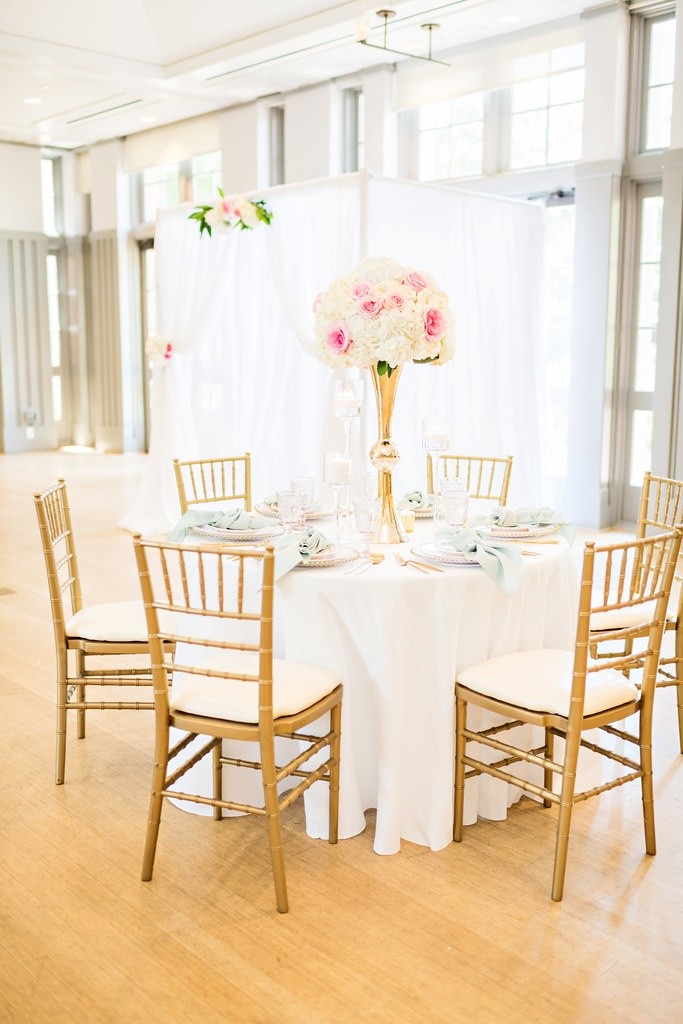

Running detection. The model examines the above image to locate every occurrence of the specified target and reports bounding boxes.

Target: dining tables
[155,485,584,856]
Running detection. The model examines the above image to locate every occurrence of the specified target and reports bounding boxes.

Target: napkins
[489,502,576,547]
[165,506,273,544]
[262,488,317,511]
[450,521,529,582]
[404,489,434,508]
[255,524,329,595]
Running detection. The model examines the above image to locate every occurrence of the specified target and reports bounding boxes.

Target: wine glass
[322,452,351,545]
[333,379,364,526]
[274,477,315,538]
[422,417,450,538]
[352,497,383,559]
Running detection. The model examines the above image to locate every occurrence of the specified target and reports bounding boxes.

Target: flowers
[308,255,456,369]
[189,186,273,236]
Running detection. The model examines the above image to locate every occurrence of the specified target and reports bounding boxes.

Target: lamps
[352,10,452,69]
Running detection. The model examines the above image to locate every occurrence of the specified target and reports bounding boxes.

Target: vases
[359,363,412,546]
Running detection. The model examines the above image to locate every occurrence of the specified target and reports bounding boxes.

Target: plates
[282,542,360,567]
[470,523,564,537]
[254,502,344,520]
[191,514,285,540]
[411,539,524,564]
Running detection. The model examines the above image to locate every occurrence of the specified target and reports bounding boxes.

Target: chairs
[425,450,514,508]
[170,449,255,519]
[448,521,683,903]
[590,471,683,756]
[129,529,348,916]
[30,480,175,789]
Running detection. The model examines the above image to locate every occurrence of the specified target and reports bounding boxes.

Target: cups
[438,478,470,526]
